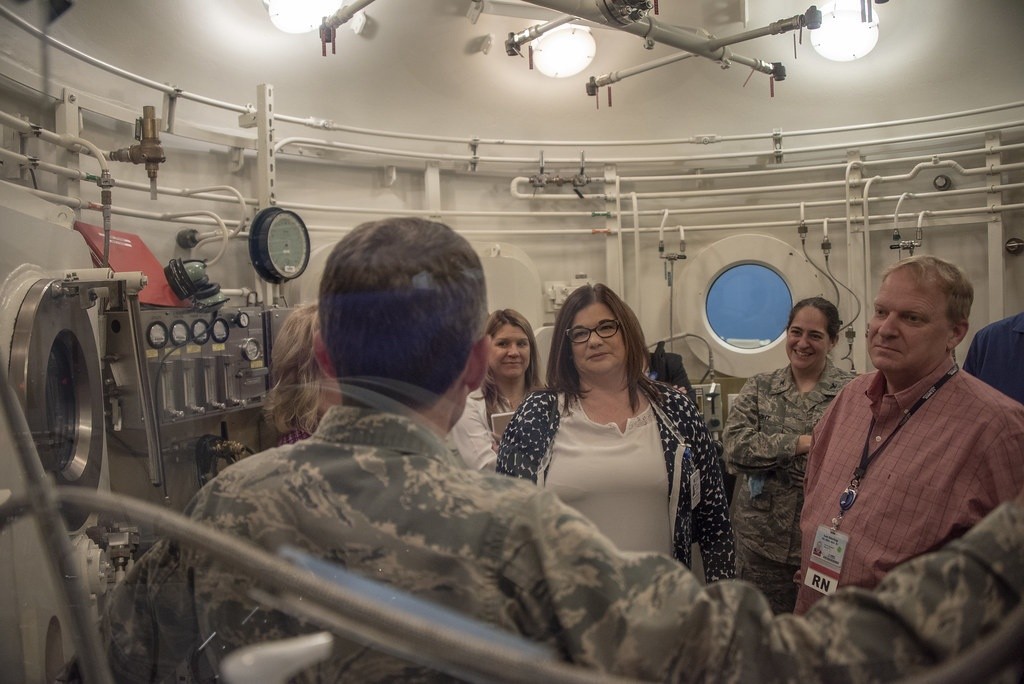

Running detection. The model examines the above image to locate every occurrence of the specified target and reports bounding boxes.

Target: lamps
[263,0,342,34]
[531,21,596,79]
[810,0,880,62]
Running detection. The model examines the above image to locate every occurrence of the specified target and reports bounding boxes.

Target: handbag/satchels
[648,341,696,402]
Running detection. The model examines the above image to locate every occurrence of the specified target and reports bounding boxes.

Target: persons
[791,255,1024,615]
[496,282,734,583]
[645,351,691,395]
[813,541,822,556]
[962,312,1024,406]
[450,309,545,470]
[60,217,1024,684]
[262,303,341,446]
[722,297,857,616]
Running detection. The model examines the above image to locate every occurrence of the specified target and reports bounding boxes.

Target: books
[74,221,193,309]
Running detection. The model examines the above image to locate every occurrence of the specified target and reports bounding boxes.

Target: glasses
[565,319,620,343]
